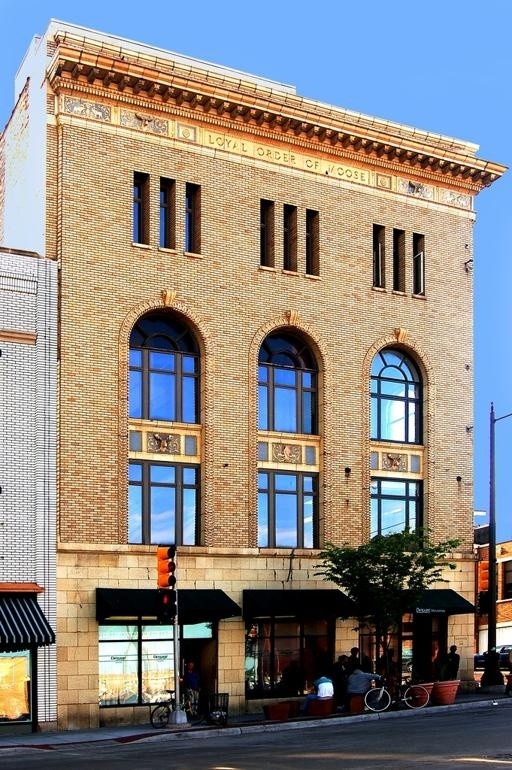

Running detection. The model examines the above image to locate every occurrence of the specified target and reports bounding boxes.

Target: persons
[185,662,199,716]
[301,645,460,717]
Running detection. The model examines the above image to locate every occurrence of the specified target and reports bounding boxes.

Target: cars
[401,648,413,672]
[474,644,512,671]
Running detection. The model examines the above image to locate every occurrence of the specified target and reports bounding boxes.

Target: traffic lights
[156,545,177,622]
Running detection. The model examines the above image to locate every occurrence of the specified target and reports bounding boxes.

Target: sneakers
[363,705,375,711]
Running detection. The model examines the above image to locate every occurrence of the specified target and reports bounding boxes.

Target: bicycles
[364,673,430,712]
[150,689,207,729]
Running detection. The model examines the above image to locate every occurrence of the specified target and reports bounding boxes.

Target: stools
[263,693,362,722]
[411,679,462,707]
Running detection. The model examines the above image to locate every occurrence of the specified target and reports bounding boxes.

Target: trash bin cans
[209,693,229,725]
